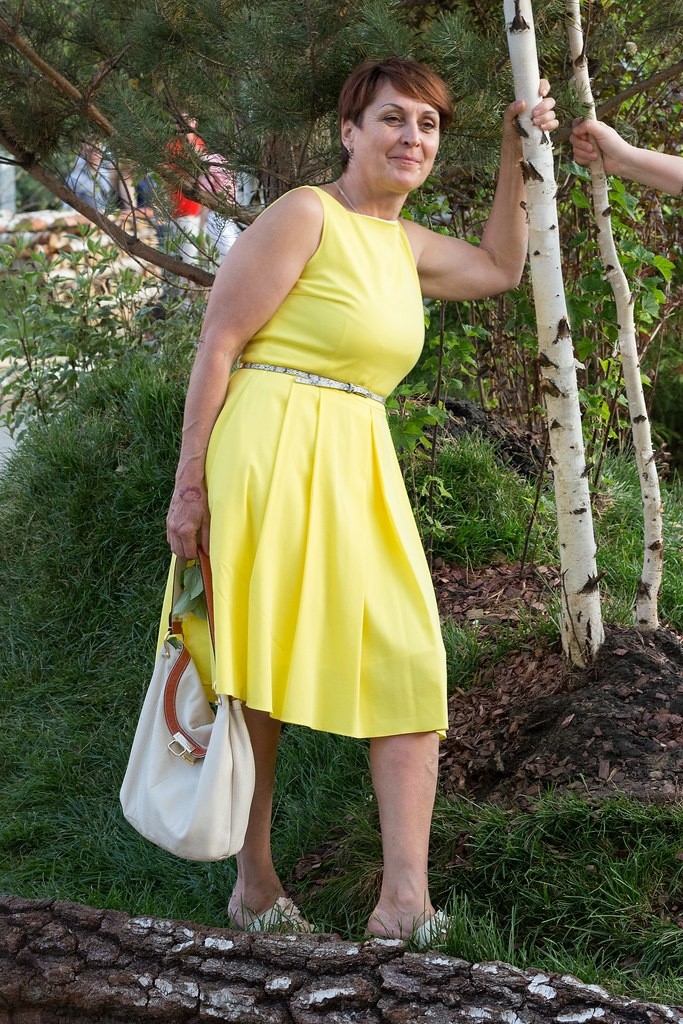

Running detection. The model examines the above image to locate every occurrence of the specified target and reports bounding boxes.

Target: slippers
[364,909,458,950]
[227,884,318,934]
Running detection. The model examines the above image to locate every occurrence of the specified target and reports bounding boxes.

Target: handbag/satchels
[120,547,257,862]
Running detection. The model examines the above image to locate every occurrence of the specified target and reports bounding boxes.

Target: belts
[239,363,385,405]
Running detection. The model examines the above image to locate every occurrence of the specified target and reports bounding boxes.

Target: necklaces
[333,181,362,215]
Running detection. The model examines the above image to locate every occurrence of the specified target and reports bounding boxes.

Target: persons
[65,109,265,305]
[167,56,560,950]
[569,117,683,201]
